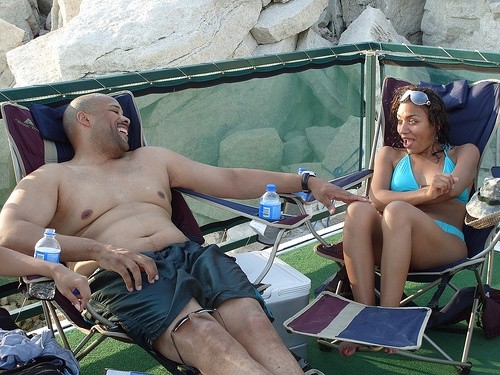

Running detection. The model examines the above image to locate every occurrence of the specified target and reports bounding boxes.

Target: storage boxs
[234,250,312,363]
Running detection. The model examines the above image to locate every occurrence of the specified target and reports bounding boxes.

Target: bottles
[257,183,281,245]
[29,228,61,300]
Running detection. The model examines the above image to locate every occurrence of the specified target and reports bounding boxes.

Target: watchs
[301,171,317,193]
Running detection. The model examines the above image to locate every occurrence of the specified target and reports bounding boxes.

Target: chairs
[278,76,500,375]
[1,90,313,375]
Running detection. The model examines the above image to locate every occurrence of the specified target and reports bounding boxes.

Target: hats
[465,177,500,218]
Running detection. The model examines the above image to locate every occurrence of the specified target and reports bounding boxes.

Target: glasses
[171,308,228,369]
[398,90,431,108]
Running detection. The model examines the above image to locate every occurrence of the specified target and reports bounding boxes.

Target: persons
[337,86,480,356]
[0,93,372,375]
[0,246,91,312]
